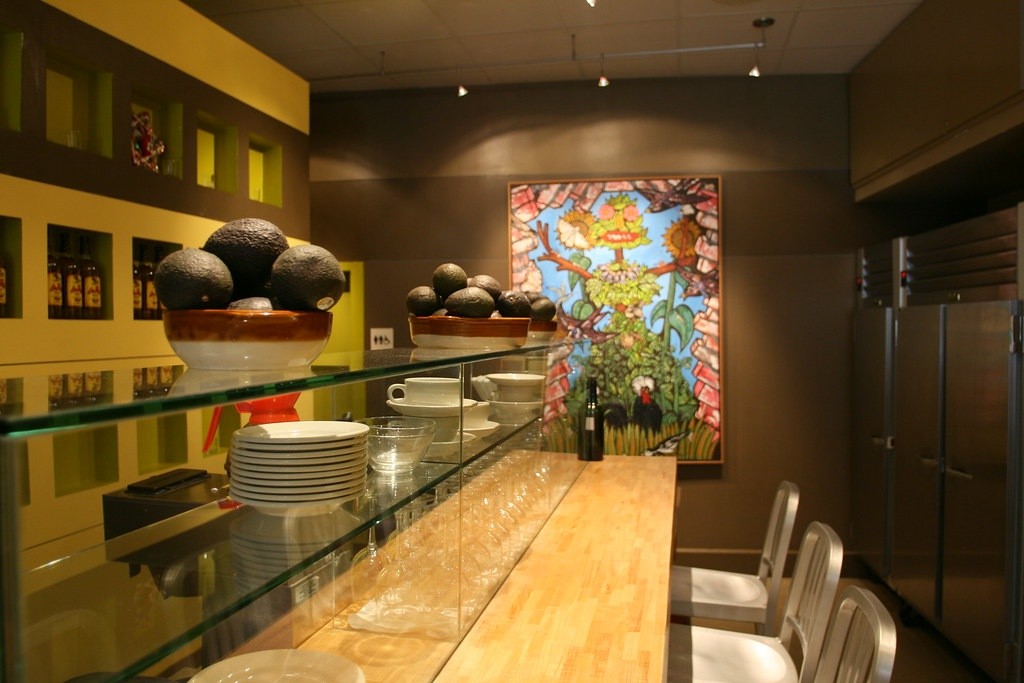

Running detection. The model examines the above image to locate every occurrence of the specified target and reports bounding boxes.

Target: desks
[217,450,578,683]
[430,449,677,681]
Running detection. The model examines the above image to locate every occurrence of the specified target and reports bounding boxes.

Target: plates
[460,421,500,437]
[186,648,368,683]
[388,433,477,458]
[486,373,546,386]
[386,398,478,417]
[227,421,370,517]
[486,401,540,414]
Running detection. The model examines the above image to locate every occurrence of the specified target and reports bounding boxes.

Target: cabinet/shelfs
[0,1,314,411]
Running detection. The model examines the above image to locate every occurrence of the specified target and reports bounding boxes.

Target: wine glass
[349,426,561,637]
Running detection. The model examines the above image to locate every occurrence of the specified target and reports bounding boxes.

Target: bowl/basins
[408,315,531,350]
[156,306,333,372]
[527,321,560,339]
[353,415,437,475]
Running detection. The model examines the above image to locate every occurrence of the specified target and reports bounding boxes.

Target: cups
[462,401,490,427]
[388,414,460,443]
[387,376,461,407]
[490,384,540,403]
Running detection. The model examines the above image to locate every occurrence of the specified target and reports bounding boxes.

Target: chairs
[671,479,799,637]
[814,583,898,683]
[666,519,845,682]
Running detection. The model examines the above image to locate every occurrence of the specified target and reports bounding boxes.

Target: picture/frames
[507,174,725,466]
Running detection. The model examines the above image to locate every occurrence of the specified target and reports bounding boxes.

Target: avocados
[407,263,556,320]
[156,218,344,310]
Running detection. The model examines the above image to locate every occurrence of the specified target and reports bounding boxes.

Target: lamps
[456,42,761,98]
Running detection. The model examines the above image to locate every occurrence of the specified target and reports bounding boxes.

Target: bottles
[0,243,13,314]
[133,256,167,320]
[0,365,179,415]
[48,250,108,320]
[576,376,604,461]
